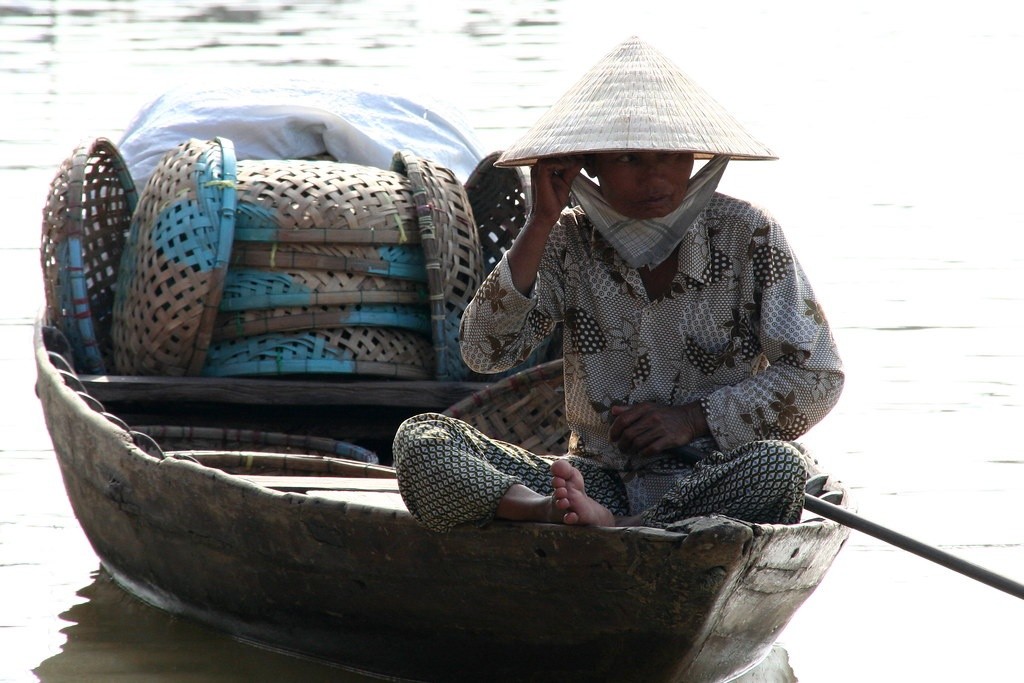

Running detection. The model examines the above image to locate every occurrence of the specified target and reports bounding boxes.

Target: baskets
[131,426,379,466]
[39,136,533,383]
[163,451,399,476]
[440,356,571,455]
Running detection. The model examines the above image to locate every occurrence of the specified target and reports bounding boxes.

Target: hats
[491,35,778,167]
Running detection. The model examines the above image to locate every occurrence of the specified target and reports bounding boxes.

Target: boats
[33,90,852,682]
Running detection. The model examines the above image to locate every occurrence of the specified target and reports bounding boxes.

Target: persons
[392,35,844,534]
